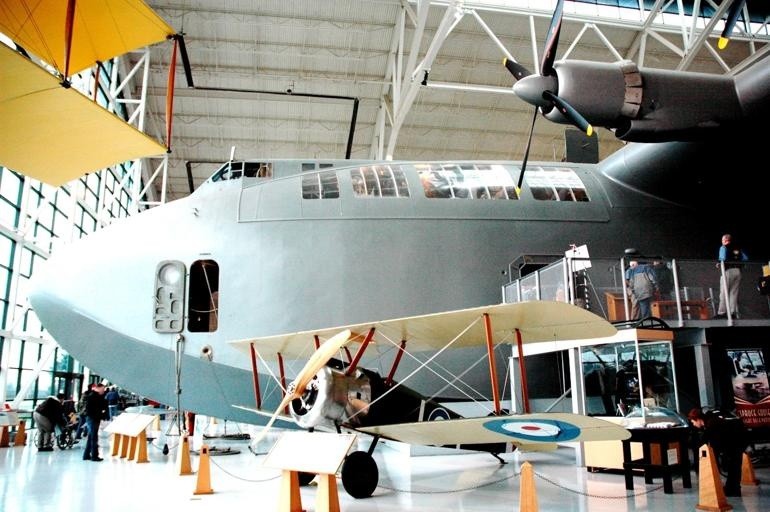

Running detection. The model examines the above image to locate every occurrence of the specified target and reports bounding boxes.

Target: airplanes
[1,0,185,189]
[27,0,770,498]
[222,296,643,496]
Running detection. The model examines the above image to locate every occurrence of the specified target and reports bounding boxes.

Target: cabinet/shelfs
[576,329,688,472]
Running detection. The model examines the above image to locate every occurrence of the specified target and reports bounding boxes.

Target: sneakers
[38,446,54,452]
[710,312,737,319]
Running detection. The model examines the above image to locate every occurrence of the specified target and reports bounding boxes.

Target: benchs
[651,298,710,322]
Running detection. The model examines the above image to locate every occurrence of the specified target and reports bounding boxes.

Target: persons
[756,260,770,318]
[652,256,674,318]
[556,280,565,302]
[30,384,166,461]
[733,352,758,389]
[626,260,658,319]
[645,382,669,407]
[689,408,750,497]
[569,272,588,308]
[626,287,640,319]
[716,233,749,318]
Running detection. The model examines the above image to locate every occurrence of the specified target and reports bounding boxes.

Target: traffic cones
[109,424,150,464]
[0,420,26,448]
[693,444,733,511]
[176,434,195,476]
[193,444,219,493]
[316,469,344,512]
[279,470,307,512]
[739,451,762,487]
[516,460,541,512]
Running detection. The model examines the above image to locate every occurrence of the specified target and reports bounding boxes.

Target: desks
[621,428,693,493]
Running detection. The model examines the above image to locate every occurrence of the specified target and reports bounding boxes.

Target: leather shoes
[83,455,103,461]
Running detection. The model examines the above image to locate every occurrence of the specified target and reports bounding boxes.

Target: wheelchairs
[34,400,83,450]
